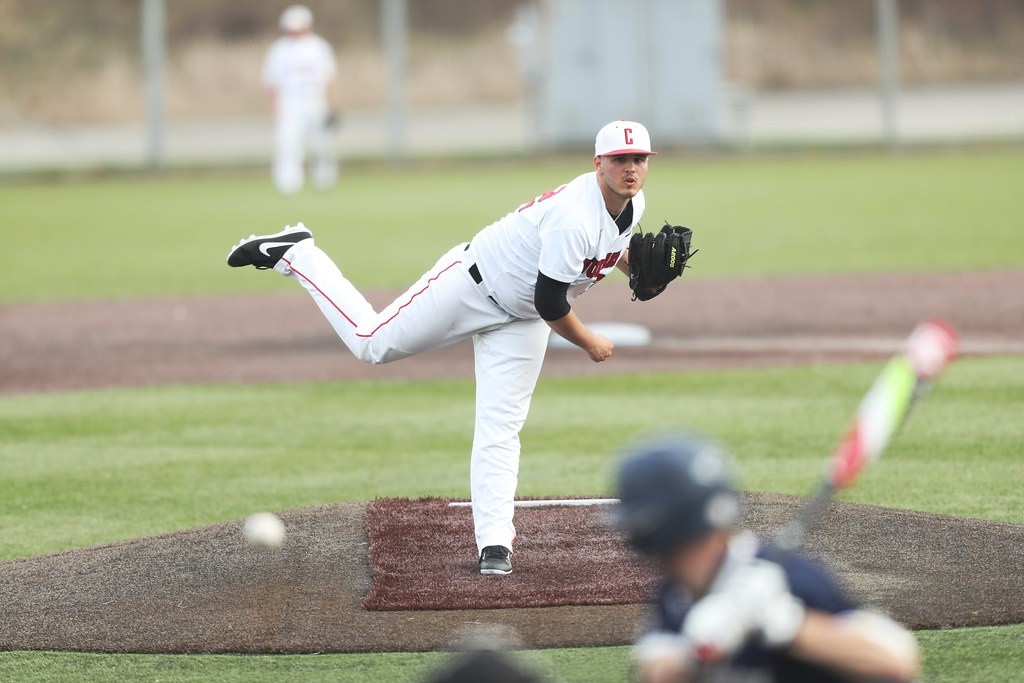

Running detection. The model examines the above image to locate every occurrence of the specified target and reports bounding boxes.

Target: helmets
[607,436,744,559]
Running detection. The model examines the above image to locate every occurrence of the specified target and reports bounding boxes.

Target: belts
[464,243,499,306]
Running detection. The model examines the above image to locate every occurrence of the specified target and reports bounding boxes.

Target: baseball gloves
[626,222,701,303]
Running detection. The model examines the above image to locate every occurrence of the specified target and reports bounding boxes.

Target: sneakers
[226,222,312,270]
[478,545,514,574]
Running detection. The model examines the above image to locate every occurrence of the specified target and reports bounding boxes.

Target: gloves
[722,561,807,650]
[679,595,747,664]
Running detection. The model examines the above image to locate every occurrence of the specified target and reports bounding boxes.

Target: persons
[227,119,698,577]
[262,7,347,191]
[612,434,923,683]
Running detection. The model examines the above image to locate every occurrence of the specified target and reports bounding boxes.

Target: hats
[594,119,657,159]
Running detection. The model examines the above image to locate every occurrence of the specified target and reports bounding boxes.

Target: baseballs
[240,511,286,555]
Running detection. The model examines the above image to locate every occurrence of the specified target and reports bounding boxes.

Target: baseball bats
[647,313,963,677]
